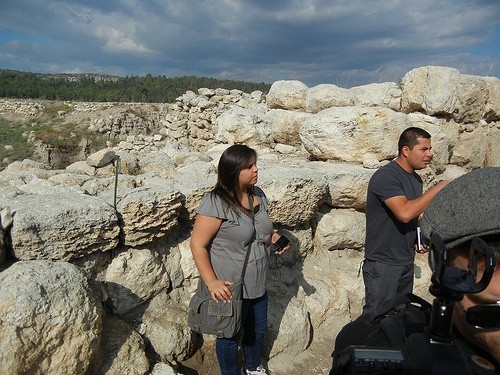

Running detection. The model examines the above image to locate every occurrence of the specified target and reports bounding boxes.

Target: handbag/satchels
[187,187,254,338]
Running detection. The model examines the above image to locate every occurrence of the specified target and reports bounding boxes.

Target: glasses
[456,300,500,331]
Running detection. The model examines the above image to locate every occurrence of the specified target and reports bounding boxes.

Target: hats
[418,167,500,252]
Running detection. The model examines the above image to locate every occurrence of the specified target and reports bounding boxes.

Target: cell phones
[417,227,429,251]
[271,235,290,255]
